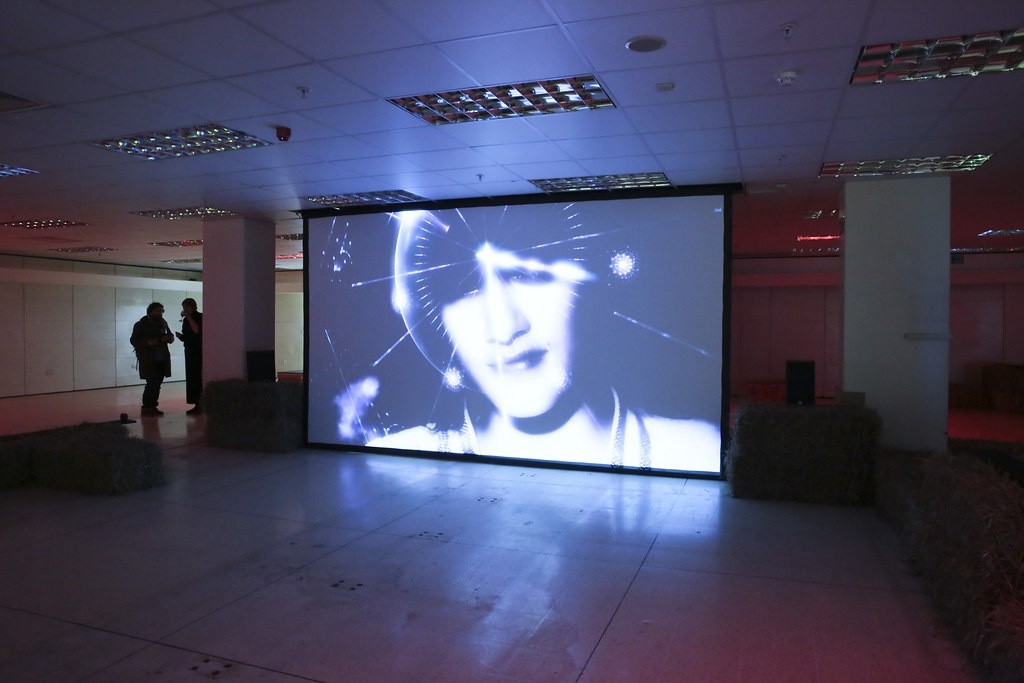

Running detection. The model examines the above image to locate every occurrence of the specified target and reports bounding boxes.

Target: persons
[175,298,203,415]
[130,302,174,415]
[368,207,721,473]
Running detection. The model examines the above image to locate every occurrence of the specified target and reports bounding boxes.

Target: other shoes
[141,406,156,417]
[186,407,202,416]
[153,407,164,415]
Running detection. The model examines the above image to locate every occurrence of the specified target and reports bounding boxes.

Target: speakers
[247,349,276,384]
[784,359,816,408]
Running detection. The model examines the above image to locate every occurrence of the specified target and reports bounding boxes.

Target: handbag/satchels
[147,343,170,373]
